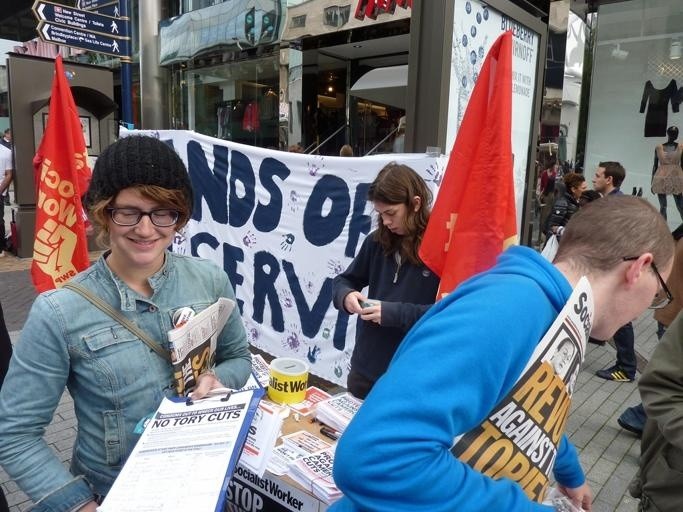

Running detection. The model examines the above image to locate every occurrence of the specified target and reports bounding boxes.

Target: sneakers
[595,365,634,382]
[618,404,648,438]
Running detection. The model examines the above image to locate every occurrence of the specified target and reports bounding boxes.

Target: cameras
[359,300,374,310]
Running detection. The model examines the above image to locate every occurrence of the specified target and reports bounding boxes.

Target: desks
[223,392,363,512]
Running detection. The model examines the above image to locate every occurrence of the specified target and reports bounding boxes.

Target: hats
[580,189,601,200]
[87,134,194,212]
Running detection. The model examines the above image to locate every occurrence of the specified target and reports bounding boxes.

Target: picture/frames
[42,112,92,148]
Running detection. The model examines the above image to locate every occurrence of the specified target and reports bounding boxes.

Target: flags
[416,27,519,301]
[29,53,93,293]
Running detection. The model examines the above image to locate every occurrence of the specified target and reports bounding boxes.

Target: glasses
[106,206,181,228]
[621,253,674,310]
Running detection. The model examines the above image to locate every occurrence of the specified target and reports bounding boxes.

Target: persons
[534,144,637,380]
[629,309,682,512]
[332,162,438,401]
[1,128,13,206]
[650,126,683,239]
[0,135,252,512]
[0,135,12,260]
[327,193,675,512]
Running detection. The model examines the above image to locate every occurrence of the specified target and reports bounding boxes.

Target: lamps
[611,44,628,60]
[670,37,683,60]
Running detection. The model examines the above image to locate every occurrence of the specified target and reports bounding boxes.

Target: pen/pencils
[308,417,342,441]
